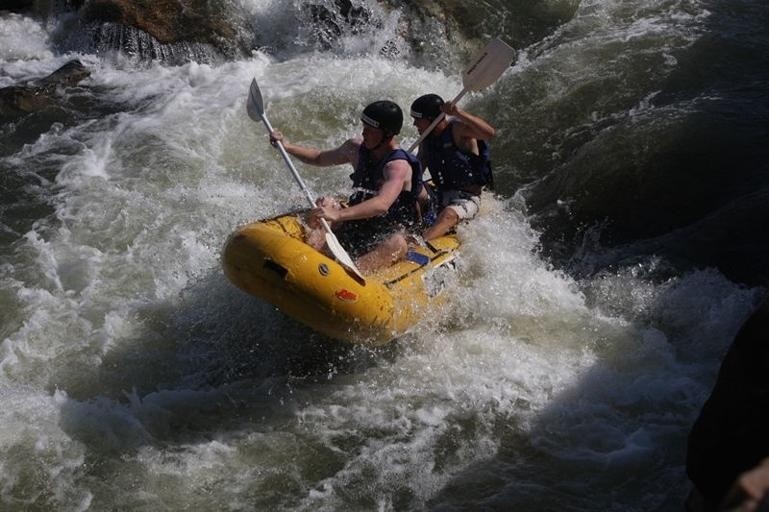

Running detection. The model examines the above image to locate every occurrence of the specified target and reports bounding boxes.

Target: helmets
[411,93,448,120]
[360,101,403,136]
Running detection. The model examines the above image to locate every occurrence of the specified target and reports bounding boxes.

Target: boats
[222,194,501,351]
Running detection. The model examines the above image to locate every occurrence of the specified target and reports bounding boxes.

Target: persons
[409,94,496,243]
[270,98,425,277]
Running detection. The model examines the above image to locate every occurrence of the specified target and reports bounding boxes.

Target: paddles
[247,78,366,287]
[406,39,516,156]
